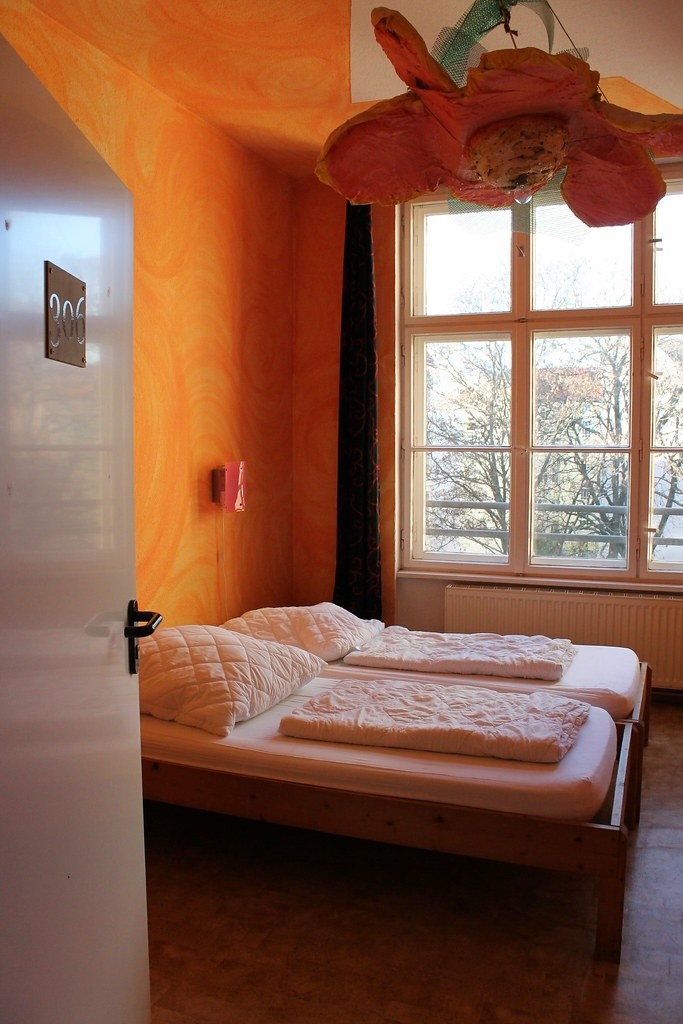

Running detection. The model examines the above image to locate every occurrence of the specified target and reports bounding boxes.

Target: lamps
[312,0,683,228]
[212,460,246,513]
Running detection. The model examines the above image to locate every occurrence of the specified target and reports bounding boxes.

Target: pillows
[220,603,386,661]
[137,624,329,735]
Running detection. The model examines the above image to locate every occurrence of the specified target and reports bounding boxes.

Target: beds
[219,601,653,748]
[137,625,636,965]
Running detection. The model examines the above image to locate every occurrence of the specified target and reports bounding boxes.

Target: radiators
[443,584,683,690]
